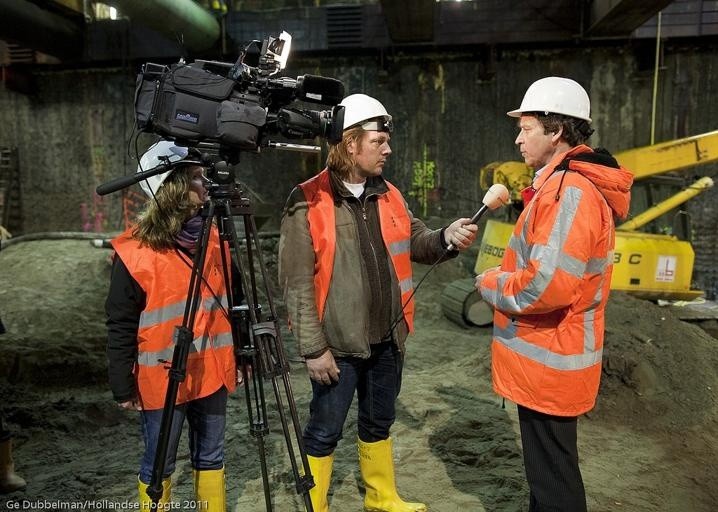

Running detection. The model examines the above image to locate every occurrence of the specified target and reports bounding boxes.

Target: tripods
[146,200,314,511]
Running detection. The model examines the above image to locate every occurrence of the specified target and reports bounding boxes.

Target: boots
[137,474,172,512]
[356,434,428,512]
[301,451,335,512]
[0,439,28,493]
[192,462,226,512]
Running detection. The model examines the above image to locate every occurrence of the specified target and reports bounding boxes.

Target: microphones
[448,184,509,251]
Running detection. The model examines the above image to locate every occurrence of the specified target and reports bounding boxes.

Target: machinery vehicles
[439,129,718,332]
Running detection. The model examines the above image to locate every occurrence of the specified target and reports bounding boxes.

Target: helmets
[136,140,188,199]
[506,76,593,125]
[326,93,393,147]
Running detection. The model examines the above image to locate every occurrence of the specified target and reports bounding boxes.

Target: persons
[104,140,255,512]
[470,74,636,512]
[279,92,480,512]
